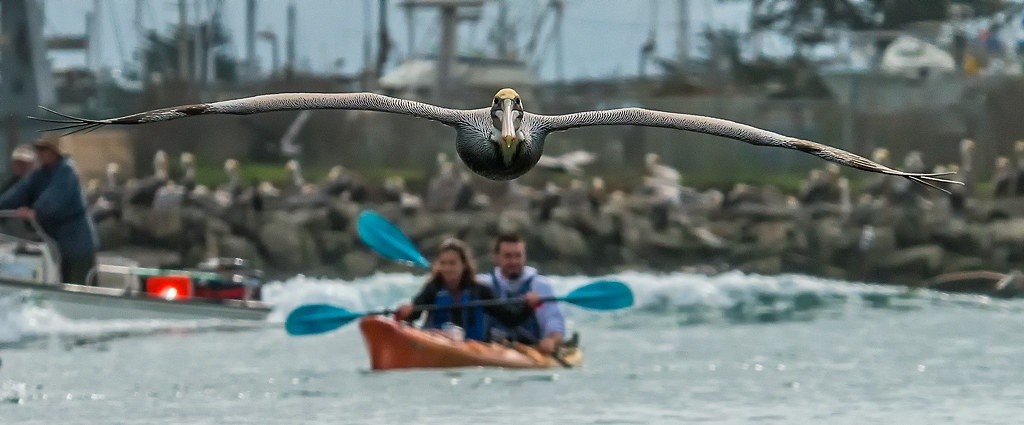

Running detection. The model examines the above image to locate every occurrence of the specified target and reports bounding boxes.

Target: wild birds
[70,110,1024,211]
[26,86,966,196]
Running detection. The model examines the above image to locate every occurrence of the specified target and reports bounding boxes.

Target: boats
[816,56,986,113]
[378,48,533,101]
[0,209,274,349]
[358,316,581,372]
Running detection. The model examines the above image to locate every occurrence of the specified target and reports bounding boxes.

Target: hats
[35,132,70,156]
[9,147,37,164]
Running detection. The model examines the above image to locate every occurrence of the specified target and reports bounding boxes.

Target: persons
[0,130,98,287]
[395,239,545,347]
[474,233,565,353]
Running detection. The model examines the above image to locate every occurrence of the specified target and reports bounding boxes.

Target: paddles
[283,279,636,338]
[354,209,577,374]
